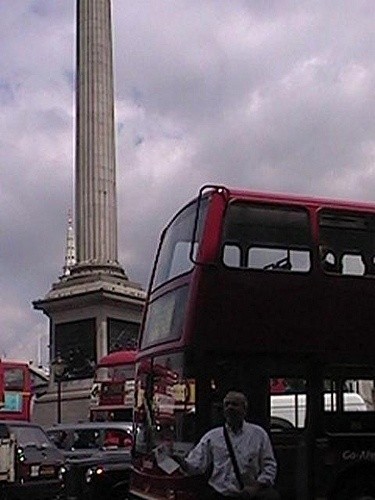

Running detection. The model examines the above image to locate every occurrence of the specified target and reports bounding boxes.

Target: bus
[0,360,48,421]
[128,179,374,499]
[90,349,139,448]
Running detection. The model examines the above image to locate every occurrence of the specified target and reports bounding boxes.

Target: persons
[162,390,278,500]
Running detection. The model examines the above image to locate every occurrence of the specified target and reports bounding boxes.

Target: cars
[0,422,141,500]
